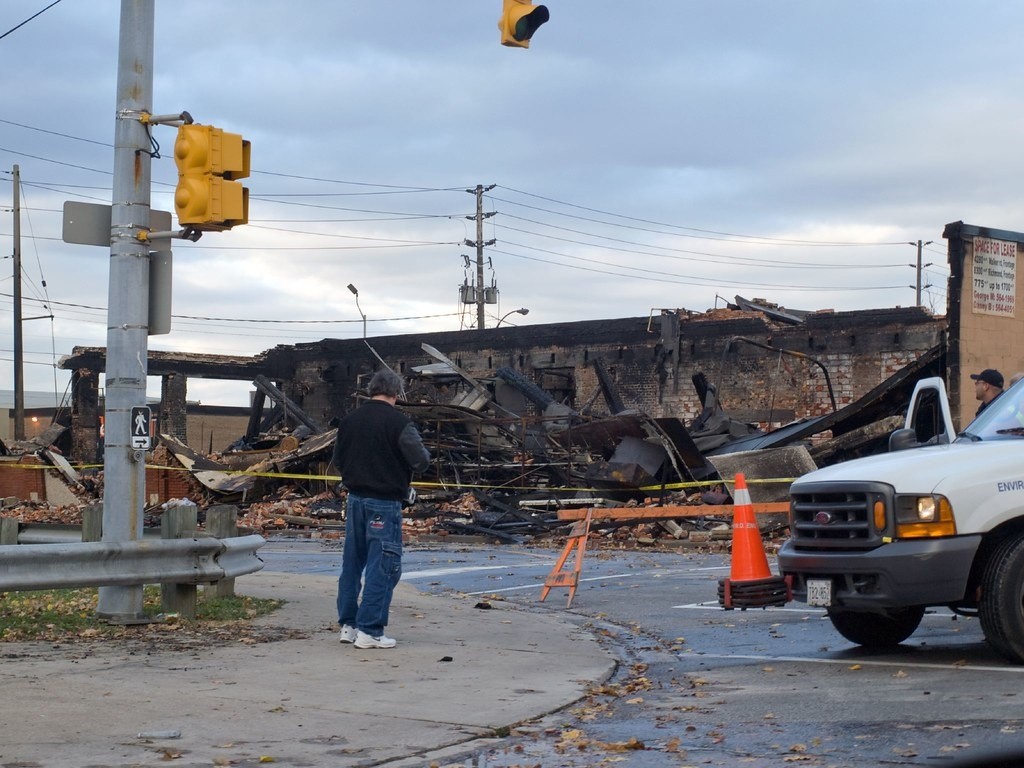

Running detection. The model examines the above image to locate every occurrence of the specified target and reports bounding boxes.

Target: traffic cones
[716,473,790,608]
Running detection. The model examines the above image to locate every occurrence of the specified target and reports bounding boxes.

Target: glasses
[975,382,986,386]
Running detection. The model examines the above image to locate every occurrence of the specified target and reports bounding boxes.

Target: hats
[970,369,1004,387]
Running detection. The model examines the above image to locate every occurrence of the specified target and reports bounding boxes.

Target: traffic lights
[496,1,550,50]
[174,124,249,233]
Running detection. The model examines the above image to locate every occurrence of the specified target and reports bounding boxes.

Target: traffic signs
[131,405,152,451]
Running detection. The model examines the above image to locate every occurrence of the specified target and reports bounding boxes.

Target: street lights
[347,283,367,339]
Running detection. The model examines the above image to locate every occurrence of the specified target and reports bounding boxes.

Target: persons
[970,369,1024,436]
[332,370,430,649]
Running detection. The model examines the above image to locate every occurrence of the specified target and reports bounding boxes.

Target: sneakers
[354,630,397,648]
[340,625,356,643]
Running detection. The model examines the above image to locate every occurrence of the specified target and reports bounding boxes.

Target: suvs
[776,372,1024,667]
